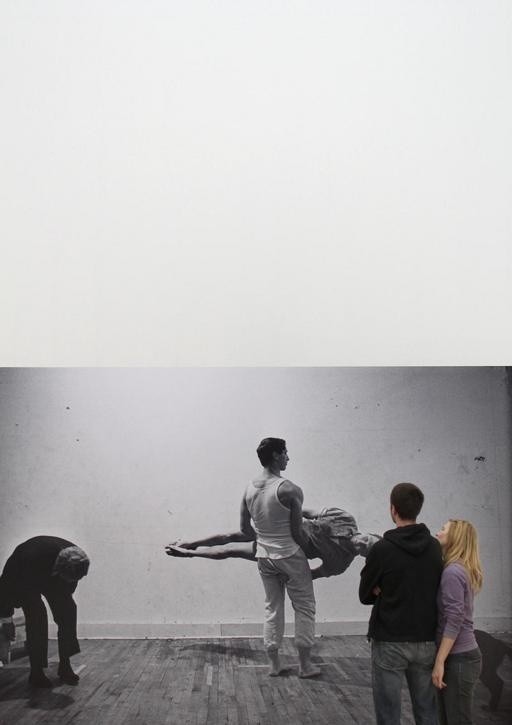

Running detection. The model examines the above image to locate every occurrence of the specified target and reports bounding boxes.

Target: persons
[431,519,484,724]
[165,507,382,580]
[359,482,444,725]
[0,535,91,690]
[238,435,324,680]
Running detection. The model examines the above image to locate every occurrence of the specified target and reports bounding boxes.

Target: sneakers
[29,668,51,688]
[58,664,79,681]
